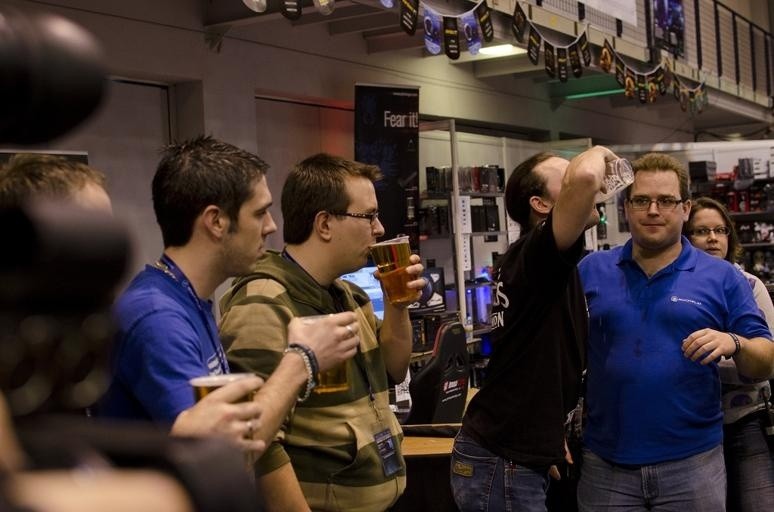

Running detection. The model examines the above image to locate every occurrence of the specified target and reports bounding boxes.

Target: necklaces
[155,260,178,283]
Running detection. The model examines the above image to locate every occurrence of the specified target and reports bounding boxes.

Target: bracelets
[283,348,313,402]
[725,331,740,360]
[287,342,320,387]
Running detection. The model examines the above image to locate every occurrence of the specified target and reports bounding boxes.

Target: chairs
[390,319,470,437]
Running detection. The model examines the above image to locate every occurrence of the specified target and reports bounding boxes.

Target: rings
[247,420,254,435]
[346,324,354,335]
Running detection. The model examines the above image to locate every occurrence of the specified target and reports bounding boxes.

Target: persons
[0,153,264,453]
[215,153,427,511]
[449,145,631,510]
[109,135,362,511]
[577,153,774,512]
[682,199,774,511]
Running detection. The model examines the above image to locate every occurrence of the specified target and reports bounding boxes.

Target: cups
[367,235,422,307]
[308,314,348,393]
[187,372,255,472]
[593,157,634,206]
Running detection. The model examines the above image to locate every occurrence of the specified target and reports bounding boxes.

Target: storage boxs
[688,159,716,185]
[739,157,767,180]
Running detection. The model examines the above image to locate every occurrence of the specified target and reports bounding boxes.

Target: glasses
[684,226,731,237]
[627,197,688,210]
[329,208,380,226]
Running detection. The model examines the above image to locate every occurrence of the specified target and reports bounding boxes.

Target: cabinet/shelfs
[418,117,593,390]
[688,181,774,300]
